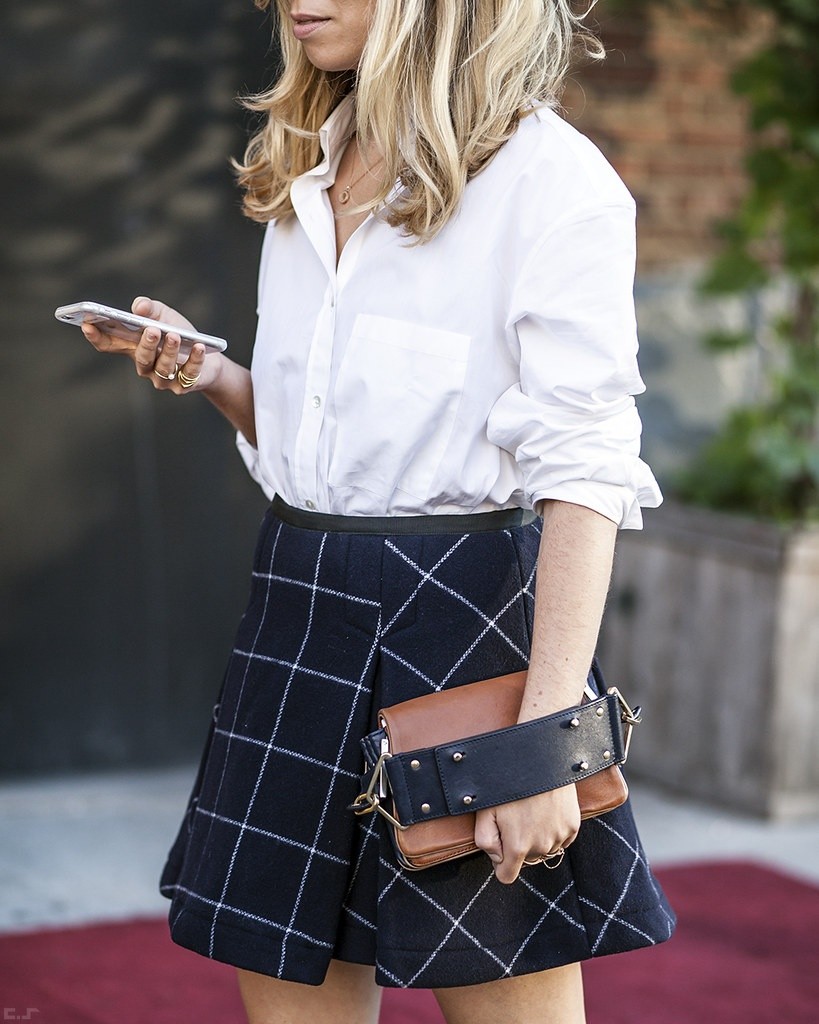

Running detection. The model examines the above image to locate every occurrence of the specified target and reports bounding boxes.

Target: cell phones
[55,301,227,355]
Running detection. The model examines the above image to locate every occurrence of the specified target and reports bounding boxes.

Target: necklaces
[339,140,385,203]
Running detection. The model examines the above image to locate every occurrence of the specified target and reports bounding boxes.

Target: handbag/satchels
[349,659,643,869]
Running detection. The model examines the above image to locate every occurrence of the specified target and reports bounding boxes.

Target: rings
[154,363,178,379]
[524,859,541,865]
[546,849,560,857]
[178,368,201,387]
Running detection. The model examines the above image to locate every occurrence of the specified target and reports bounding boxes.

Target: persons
[81,1,680,1023]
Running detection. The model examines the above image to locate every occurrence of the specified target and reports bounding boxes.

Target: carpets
[2,850,819,1024]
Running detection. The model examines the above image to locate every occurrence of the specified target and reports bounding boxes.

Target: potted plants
[588,1,817,822]
